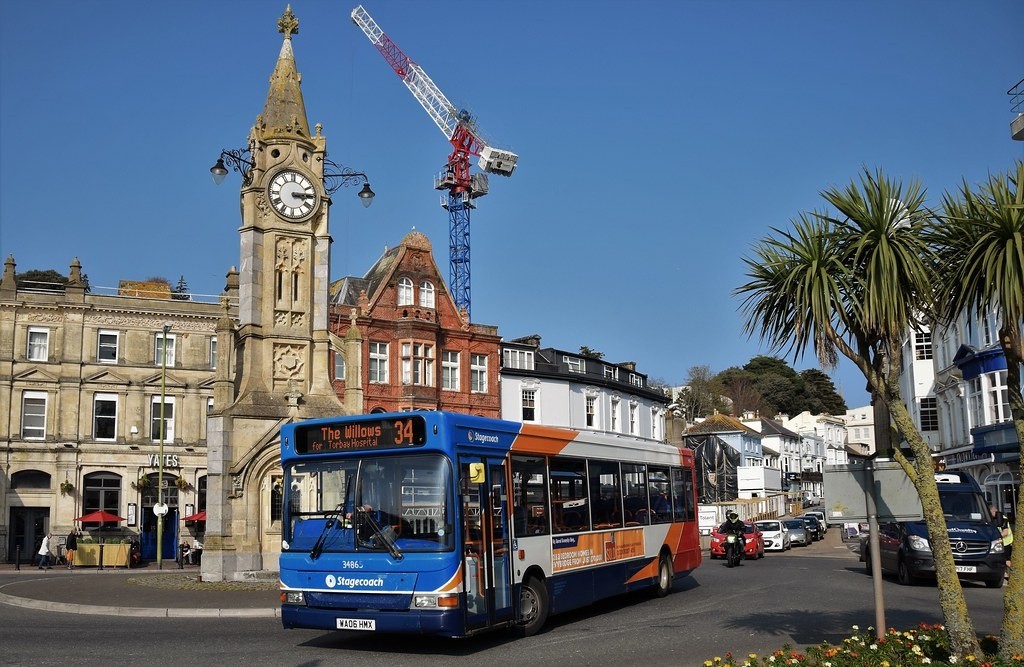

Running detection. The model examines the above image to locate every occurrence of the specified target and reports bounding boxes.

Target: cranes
[349,3,521,325]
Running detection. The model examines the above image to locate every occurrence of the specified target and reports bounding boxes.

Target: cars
[711,504,830,560]
[804,491,821,507]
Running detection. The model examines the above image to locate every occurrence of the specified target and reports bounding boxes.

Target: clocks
[270,170,316,219]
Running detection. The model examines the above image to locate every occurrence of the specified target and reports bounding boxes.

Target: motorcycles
[721,529,742,568]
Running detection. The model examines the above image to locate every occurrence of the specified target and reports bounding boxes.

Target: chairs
[553,507,657,532]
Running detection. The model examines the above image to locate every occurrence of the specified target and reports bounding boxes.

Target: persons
[523,487,687,533]
[66,528,77,569]
[343,469,391,544]
[179,541,191,565]
[986,504,1014,581]
[716,509,745,560]
[38,533,53,569]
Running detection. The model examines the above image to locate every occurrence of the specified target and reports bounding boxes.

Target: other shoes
[68,567,71,569]
[71,565,75,568]
[47,566,53,568]
[740,551,744,559]
[39,566,44,569]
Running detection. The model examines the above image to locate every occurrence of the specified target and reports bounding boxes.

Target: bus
[277,411,702,640]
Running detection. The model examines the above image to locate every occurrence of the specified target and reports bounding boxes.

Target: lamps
[209,139,256,188]
[323,152,376,209]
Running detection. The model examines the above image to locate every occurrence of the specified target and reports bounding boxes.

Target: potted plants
[103,538,131,569]
[72,538,103,568]
[60,483,74,497]
[175,475,187,491]
[139,474,151,487]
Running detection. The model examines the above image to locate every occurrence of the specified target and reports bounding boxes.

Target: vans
[859,469,1006,588]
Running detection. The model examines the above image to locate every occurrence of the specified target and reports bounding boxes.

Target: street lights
[152,321,174,571]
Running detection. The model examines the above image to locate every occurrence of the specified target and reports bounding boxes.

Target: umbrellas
[178,511,207,522]
[73,509,127,565]
[129,536,141,568]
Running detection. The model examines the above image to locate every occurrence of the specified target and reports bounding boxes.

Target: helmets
[729,511,738,522]
